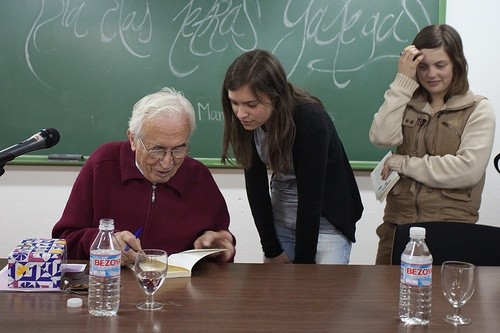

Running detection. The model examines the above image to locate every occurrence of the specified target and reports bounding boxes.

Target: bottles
[88,218,122,317]
[398,226,433,324]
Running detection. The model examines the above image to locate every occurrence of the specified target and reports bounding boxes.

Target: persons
[52,87,237,262]
[369,24,496,265]
[222,49,365,264]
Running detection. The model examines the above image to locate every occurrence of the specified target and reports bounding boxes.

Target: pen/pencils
[125,226,143,252]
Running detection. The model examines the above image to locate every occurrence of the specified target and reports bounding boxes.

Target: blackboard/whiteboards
[0,0,447,169]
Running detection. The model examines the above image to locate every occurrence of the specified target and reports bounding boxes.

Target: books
[370,150,401,203]
[127,249,228,279]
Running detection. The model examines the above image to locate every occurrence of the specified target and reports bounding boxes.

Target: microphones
[0,128,61,161]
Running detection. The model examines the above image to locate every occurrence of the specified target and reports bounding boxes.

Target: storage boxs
[8,238,67,288]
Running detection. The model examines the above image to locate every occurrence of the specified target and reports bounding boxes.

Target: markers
[48,154,83,161]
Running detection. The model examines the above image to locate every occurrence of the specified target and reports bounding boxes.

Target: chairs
[391,221,500,267]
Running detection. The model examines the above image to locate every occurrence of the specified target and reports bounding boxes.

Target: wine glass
[134,249,168,311]
[440,260,476,326]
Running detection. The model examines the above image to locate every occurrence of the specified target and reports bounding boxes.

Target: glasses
[140,138,191,160]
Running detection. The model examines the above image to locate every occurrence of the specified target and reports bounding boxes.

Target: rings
[400,52,403,56]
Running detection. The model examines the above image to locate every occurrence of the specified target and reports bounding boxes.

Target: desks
[0,258,500,333]
